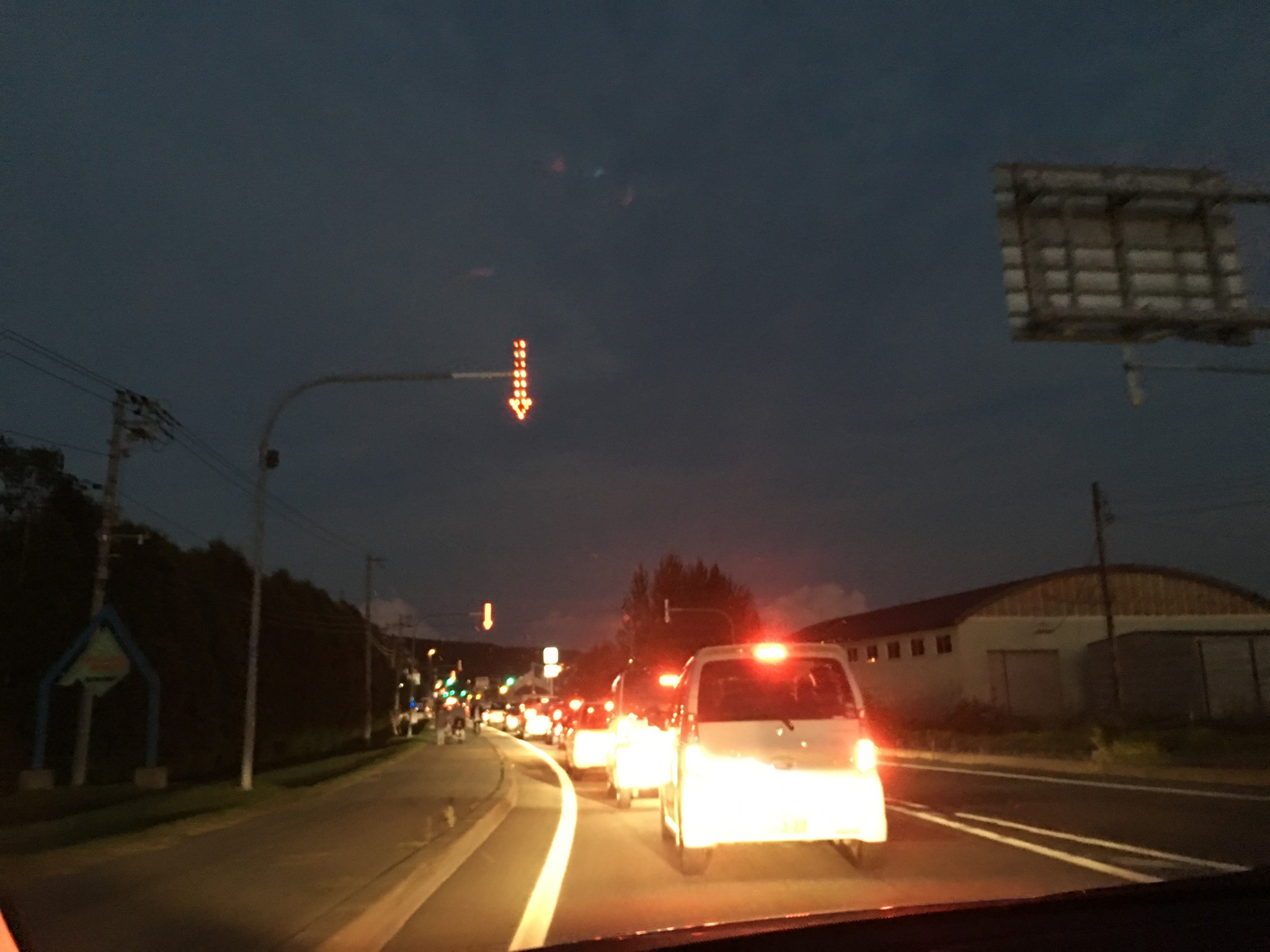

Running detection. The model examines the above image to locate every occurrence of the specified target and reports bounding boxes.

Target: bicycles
[473,720,482,735]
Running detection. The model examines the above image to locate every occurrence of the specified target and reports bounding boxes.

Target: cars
[481,692,617,780]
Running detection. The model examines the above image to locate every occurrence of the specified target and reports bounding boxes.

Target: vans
[605,663,682,812]
[658,639,887,867]
